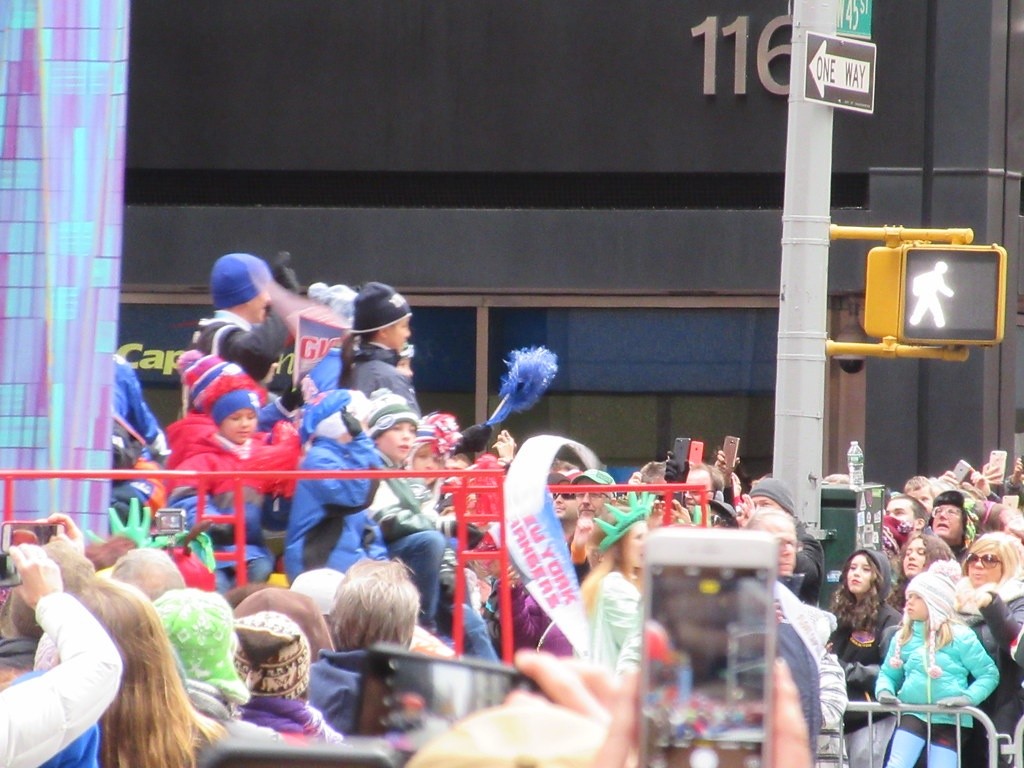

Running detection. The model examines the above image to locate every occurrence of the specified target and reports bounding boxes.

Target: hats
[547,473,570,484]
[889,559,963,676]
[212,389,259,424]
[155,589,250,704]
[176,350,268,414]
[929,489,978,549]
[403,412,462,478]
[290,569,344,614]
[881,516,912,557]
[571,469,617,497]
[308,282,357,322]
[211,253,270,308]
[366,388,419,439]
[749,477,794,516]
[353,282,413,333]
[231,611,311,698]
[314,389,371,438]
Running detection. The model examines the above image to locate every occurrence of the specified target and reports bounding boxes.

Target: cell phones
[989,450,1007,481]
[952,459,972,484]
[1,521,67,553]
[673,437,691,472]
[689,442,704,464]
[352,643,545,741]
[722,435,739,468]
[638,527,781,768]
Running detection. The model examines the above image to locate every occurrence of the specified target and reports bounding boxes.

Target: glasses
[966,553,1001,569]
[932,507,962,519]
[779,540,804,552]
[552,492,575,499]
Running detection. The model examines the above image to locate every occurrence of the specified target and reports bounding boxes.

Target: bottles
[847,440,865,487]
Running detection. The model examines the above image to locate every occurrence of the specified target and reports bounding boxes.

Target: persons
[0,511,463,768]
[108,253,492,661]
[405,430,1024,768]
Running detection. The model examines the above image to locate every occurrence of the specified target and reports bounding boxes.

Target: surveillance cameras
[834,325,870,374]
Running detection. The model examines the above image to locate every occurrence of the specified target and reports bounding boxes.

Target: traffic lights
[864,245,1007,345]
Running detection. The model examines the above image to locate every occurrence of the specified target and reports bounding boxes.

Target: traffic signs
[802,30,876,115]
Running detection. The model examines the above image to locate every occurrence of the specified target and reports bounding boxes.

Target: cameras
[156,508,187,535]
